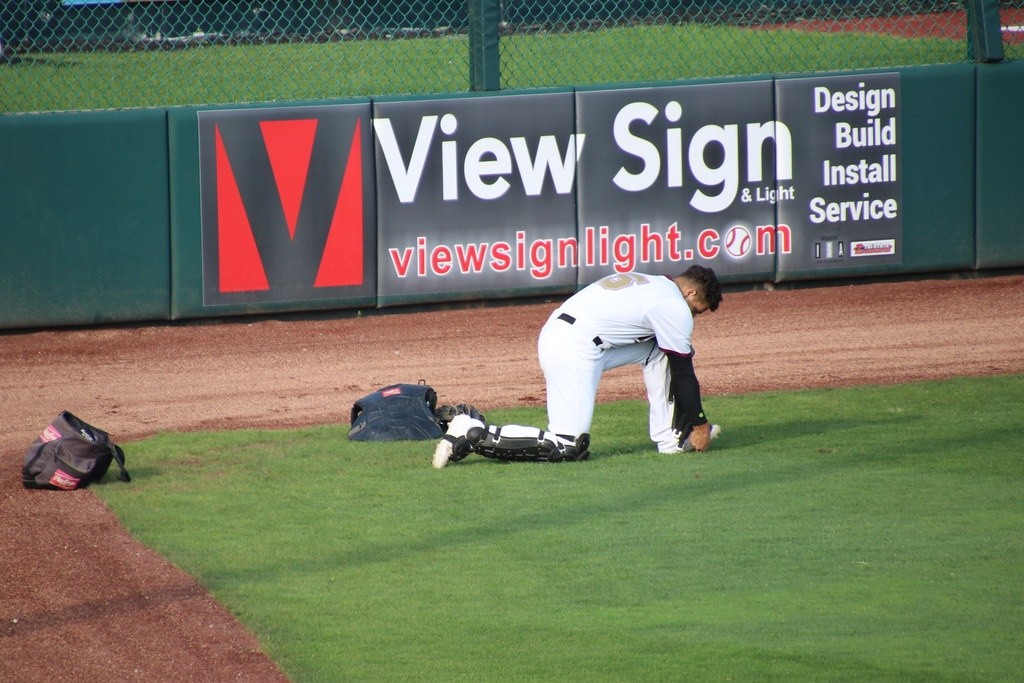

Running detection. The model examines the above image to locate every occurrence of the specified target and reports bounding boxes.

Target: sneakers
[657,423,722,455]
[429,414,473,469]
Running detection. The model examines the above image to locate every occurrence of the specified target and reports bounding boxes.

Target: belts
[557,313,605,352]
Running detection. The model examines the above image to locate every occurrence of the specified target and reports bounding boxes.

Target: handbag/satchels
[21,411,131,492]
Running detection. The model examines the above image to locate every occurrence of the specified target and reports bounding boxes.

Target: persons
[431,264,723,470]
